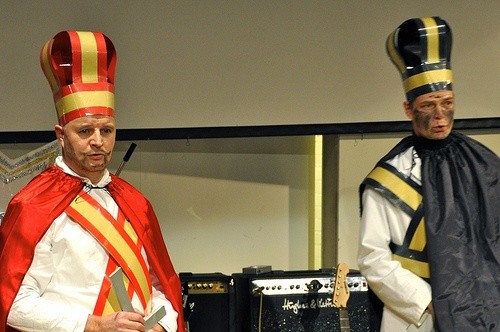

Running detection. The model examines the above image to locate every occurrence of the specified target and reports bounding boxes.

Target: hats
[384,12,456,101]
[39,27,119,123]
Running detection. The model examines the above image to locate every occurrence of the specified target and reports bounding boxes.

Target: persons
[355,17,500,332]
[1,30,190,332]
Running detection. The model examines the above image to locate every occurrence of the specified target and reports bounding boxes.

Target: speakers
[229,268,371,332]
[176,270,238,332]
